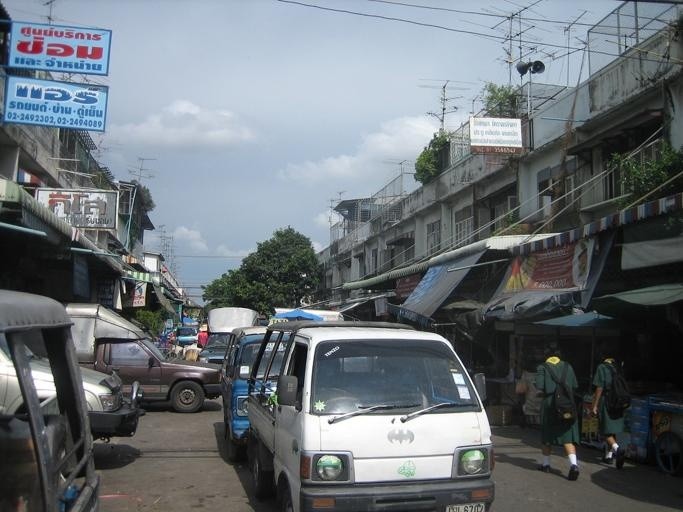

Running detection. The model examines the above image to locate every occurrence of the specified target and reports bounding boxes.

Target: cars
[175,327,198,345]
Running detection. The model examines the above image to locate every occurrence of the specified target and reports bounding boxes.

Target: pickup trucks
[247,321,494,512]
[219,326,291,461]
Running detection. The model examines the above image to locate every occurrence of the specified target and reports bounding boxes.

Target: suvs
[0,333,145,443]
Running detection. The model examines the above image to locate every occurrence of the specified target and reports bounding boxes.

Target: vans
[0,290,99,511]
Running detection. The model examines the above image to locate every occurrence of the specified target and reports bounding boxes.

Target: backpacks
[541,361,576,425]
[602,362,631,410]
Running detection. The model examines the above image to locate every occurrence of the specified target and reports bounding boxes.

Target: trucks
[64,303,222,412]
[197,307,258,363]
[255,307,343,325]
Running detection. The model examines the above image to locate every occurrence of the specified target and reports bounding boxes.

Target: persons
[591,341,628,470]
[196,324,207,344]
[533,346,582,481]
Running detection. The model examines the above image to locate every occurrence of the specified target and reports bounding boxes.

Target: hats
[198,324,208,332]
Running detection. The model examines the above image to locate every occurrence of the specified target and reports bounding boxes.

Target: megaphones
[516,61,528,74]
[530,61,546,76]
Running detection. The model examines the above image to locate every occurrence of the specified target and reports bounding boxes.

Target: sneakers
[538,464,550,473]
[568,465,579,480]
[602,456,613,464]
[616,447,625,468]
